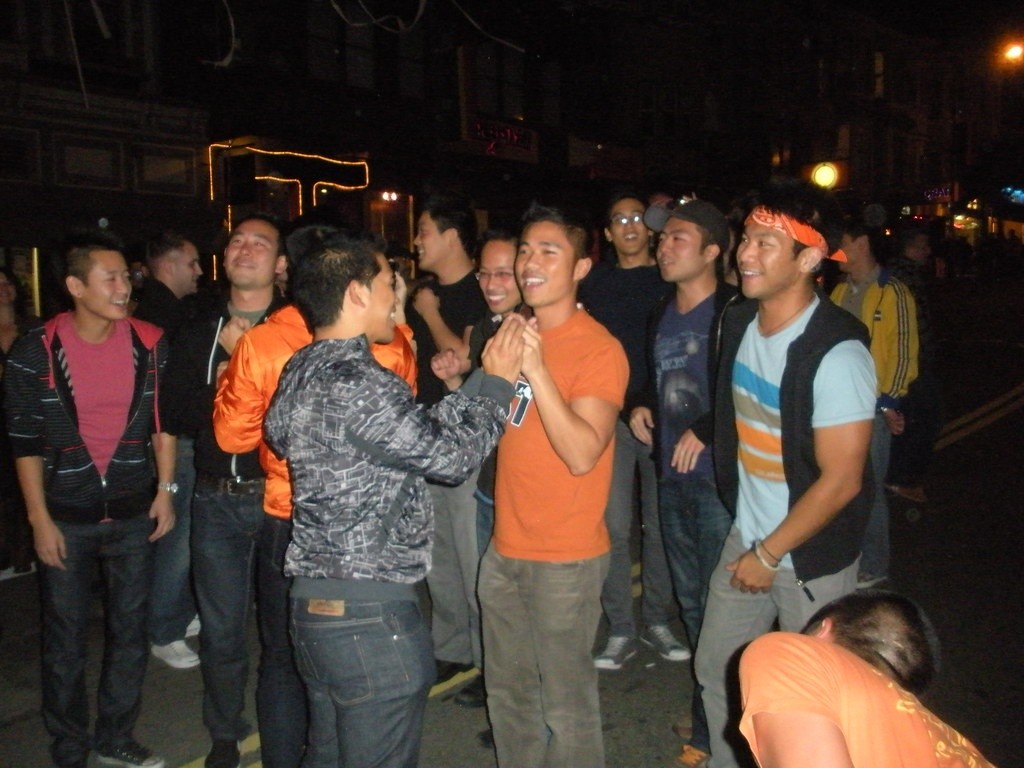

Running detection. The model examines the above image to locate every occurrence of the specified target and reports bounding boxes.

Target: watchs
[157,482,179,496]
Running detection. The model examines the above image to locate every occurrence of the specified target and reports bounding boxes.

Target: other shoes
[436,661,459,684]
[476,729,495,748]
[675,745,709,767]
[455,676,487,707]
[204,740,241,768]
[0,560,37,581]
[883,481,924,501]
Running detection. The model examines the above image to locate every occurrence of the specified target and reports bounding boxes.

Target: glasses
[473,271,511,283]
[613,213,643,225]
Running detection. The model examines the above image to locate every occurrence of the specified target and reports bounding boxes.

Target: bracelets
[751,539,780,572]
[759,538,782,562]
[880,407,887,412]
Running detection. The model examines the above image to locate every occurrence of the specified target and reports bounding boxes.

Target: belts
[197,475,265,495]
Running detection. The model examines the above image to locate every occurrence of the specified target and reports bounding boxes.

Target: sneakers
[185,614,200,637]
[98,739,164,768]
[151,640,200,668]
[593,635,636,670]
[640,623,692,662]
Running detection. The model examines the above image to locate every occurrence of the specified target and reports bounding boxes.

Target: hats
[644,201,730,250]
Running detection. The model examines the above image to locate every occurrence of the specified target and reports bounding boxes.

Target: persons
[405,187,486,708]
[619,197,742,768]
[0,172,1024,768]
[828,222,919,594]
[211,225,420,768]
[261,229,527,768]
[476,197,631,768]
[430,223,522,747]
[737,589,997,768]
[695,172,877,768]
[160,213,289,768]
[588,192,691,670]
[0,229,180,768]
